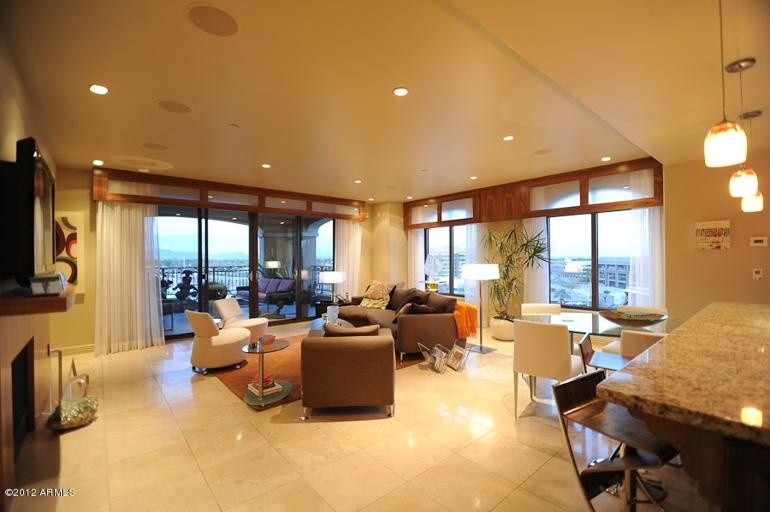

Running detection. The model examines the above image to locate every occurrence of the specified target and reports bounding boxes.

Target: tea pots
[327,306,339,324]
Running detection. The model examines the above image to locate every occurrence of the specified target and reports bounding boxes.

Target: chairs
[578,332,635,375]
[520,303,562,316]
[213,298,269,349]
[619,328,670,359]
[184,309,251,377]
[552,370,679,512]
[512,319,582,420]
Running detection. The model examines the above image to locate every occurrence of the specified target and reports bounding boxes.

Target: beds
[594,301,770,512]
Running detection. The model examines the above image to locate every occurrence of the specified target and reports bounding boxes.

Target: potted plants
[478,222,553,342]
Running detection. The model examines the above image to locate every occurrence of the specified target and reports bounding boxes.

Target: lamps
[703,0,749,170]
[295,270,310,288]
[264,260,281,275]
[737,111,765,215]
[461,263,500,355]
[319,270,348,302]
[725,56,759,199]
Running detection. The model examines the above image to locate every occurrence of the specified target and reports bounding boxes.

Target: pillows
[257,279,269,293]
[323,323,380,338]
[406,288,432,304]
[426,291,457,314]
[393,291,422,314]
[357,295,390,311]
[363,280,397,300]
[407,302,447,315]
[277,279,295,292]
[392,302,428,325]
[387,287,413,310]
[265,278,281,293]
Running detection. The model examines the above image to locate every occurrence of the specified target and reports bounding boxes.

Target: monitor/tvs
[0,136,58,290]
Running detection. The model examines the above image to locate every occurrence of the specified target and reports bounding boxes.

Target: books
[247,381,283,397]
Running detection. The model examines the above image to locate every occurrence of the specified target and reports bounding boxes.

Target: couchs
[299,327,396,419]
[236,286,295,315]
[337,295,457,362]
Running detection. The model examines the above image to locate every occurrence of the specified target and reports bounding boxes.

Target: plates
[598,310,668,326]
[257,334,276,345]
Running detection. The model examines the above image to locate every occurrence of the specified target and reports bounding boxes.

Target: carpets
[212,334,428,413]
